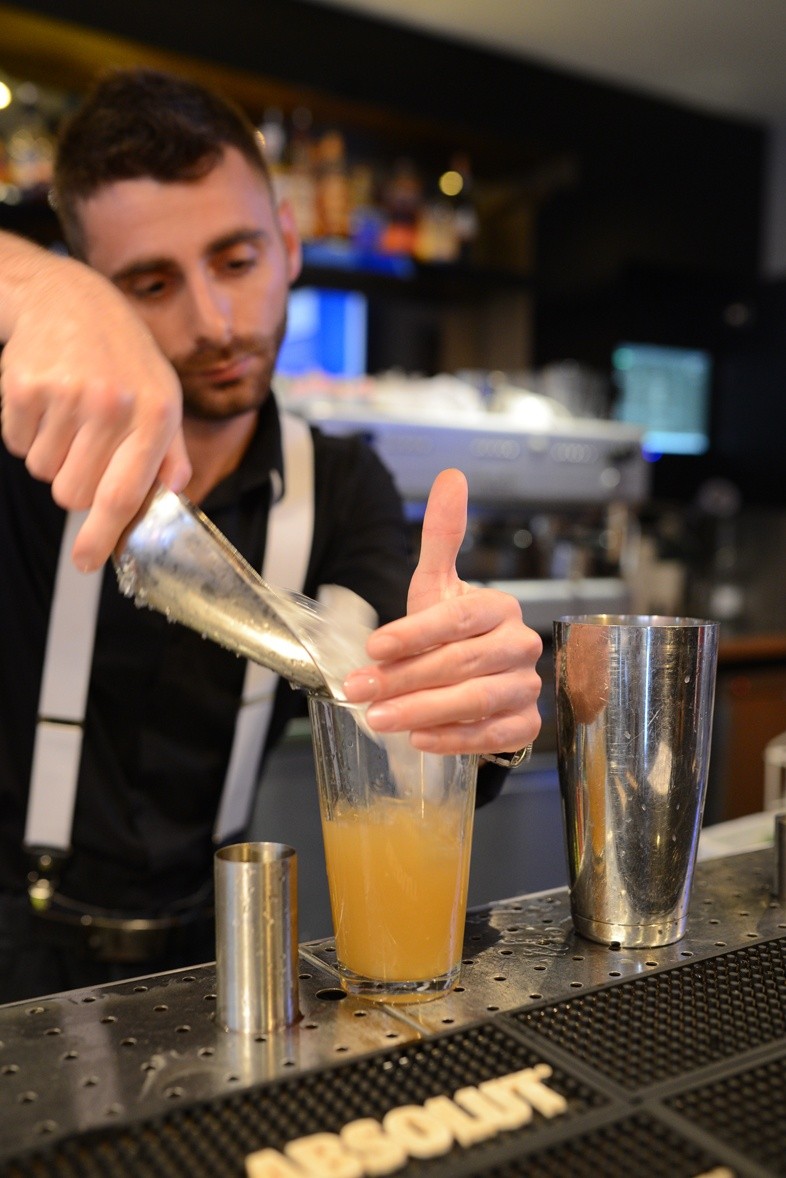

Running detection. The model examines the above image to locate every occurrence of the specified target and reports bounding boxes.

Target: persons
[0,74,542,1008]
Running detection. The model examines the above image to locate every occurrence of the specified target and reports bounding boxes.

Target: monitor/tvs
[275,264,380,381]
[615,341,714,456]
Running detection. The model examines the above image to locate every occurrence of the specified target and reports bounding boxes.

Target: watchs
[477,740,534,769]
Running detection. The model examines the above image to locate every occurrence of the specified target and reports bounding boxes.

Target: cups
[305,683,476,1001]
[553,611,720,948]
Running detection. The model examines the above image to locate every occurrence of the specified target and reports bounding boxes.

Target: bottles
[0,69,481,269]
